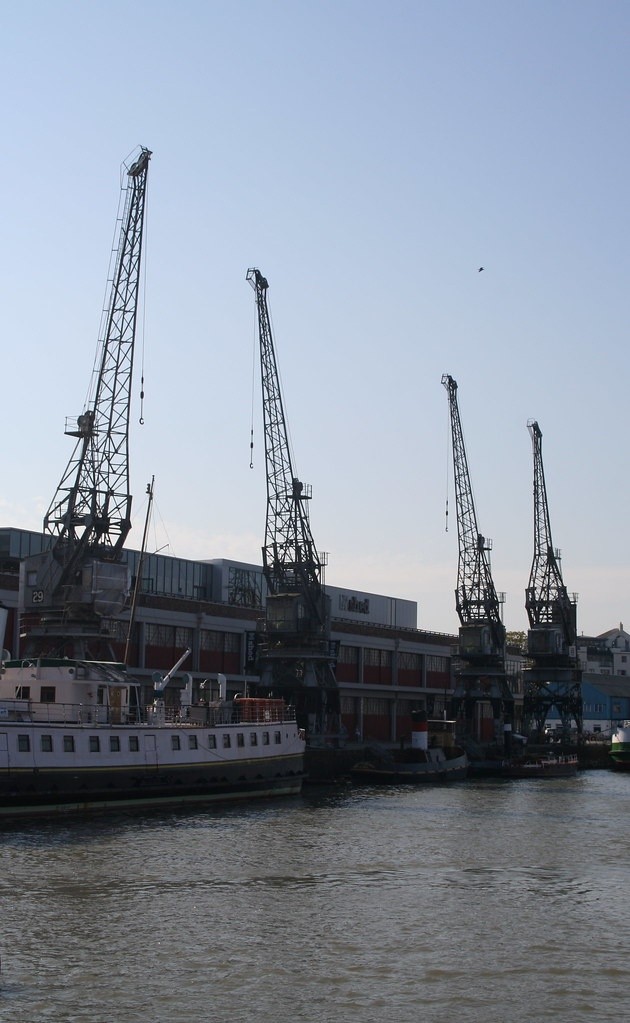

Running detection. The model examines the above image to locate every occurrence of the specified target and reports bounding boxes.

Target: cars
[544,727,578,745]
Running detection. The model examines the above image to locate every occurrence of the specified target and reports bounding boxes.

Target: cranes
[240,268,345,788]
[441,374,519,780]
[16,139,153,664]
[524,422,586,773]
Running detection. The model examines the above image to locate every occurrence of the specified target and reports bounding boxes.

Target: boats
[610,726,630,767]
[353,743,468,782]
[0,658,305,818]
